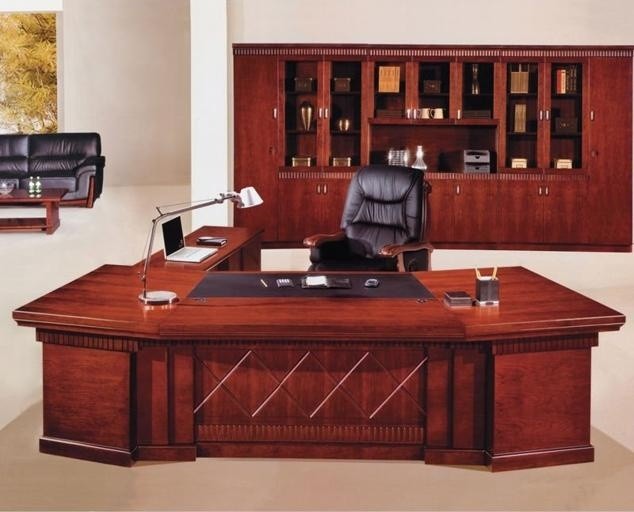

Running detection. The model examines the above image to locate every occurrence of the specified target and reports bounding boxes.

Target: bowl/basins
[0,181,14,194]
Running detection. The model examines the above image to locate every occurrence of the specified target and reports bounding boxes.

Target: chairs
[303,162,432,272]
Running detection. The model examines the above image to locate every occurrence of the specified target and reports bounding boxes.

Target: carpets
[1,400,634,511]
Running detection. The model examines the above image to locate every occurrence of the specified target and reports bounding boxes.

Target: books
[299,274,353,291]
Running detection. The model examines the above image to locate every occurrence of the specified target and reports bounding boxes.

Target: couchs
[1,134,105,209]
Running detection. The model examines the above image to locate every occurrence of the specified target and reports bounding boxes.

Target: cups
[430,108,444,120]
[416,108,430,120]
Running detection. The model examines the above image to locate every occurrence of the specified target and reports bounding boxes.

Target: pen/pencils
[261,278,268,287]
[492,266,498,278]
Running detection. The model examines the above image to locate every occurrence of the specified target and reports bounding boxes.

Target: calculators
[276,278,294,287]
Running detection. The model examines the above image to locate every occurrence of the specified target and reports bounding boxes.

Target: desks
[12,265,625,472]
[132,225,263,273]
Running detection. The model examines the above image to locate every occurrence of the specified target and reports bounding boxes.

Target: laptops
[161,215,218,263]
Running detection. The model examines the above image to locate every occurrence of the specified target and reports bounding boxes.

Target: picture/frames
[0,11,66,133]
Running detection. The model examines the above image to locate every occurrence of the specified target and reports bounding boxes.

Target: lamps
[138,184,263,310]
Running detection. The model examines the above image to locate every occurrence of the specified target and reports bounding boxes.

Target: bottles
[411,145,427,171]
[27,175,42,194]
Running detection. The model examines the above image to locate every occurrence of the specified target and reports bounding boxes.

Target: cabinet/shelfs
[278,42,368,172]
[497,172,591,252]
[278,172,356,246]
[501,43,588,171]
[424,177,497,251]
[368,43,501,176]
[233,41,278,246]
[588,43,634,253]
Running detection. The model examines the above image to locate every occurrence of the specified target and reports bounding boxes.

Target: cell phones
[364,279,379,287]
